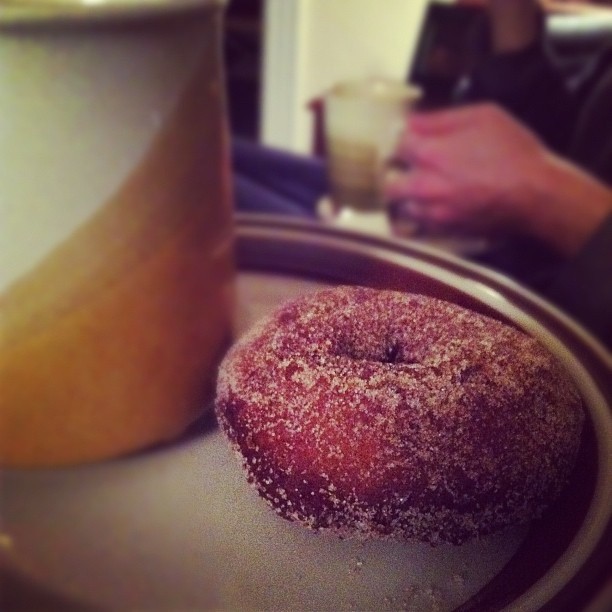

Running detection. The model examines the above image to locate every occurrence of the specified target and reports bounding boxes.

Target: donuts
[210,282,599,551]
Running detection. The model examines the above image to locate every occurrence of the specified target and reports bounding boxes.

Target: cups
[321,78,423,211]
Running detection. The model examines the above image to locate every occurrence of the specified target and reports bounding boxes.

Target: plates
[0,210,611,612]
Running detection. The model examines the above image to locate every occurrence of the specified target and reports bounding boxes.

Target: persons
[382,2,611,349]
